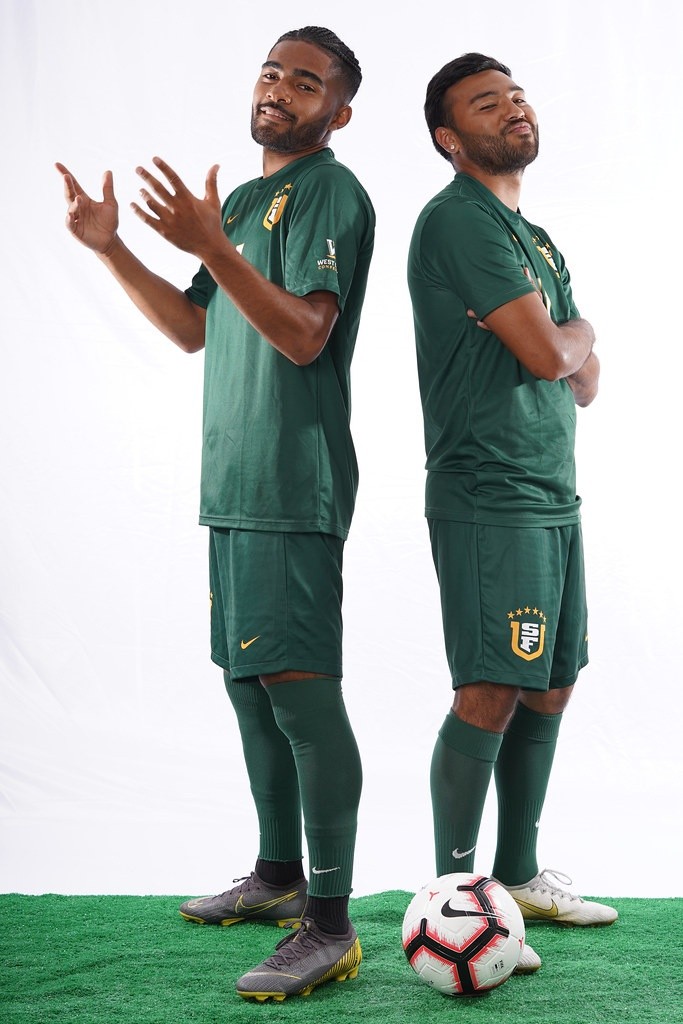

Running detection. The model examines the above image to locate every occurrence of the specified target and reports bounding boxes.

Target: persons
[58,26,380,1002]
[407,52,621,975]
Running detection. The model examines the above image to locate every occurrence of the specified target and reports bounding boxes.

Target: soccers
[401,873,525,997]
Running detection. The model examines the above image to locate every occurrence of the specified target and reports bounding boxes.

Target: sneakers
[178,871,308,929]
[487,872,619,926]
[512,944,541,974]
[234,917,362,1002]
